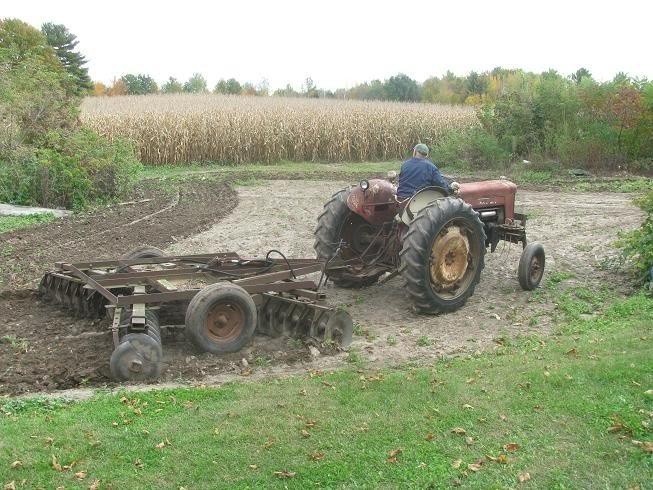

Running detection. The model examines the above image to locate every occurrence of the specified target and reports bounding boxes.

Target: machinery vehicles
[311,171,545,316]
